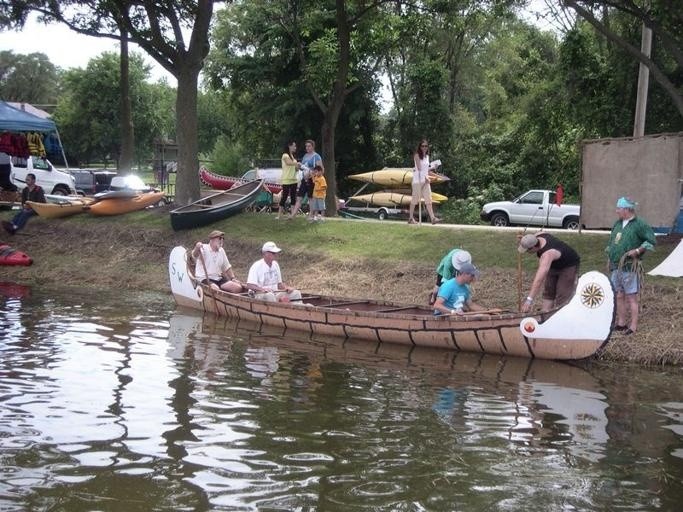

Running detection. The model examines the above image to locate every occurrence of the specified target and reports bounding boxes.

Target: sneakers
[623,329,637,334]
[408,218,418,224]
[1,220,18,235]
[432,218,440,225]
[612,324,628,331]
[431,292,437,303]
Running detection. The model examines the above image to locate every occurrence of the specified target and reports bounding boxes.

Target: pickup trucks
[481,188,582,229]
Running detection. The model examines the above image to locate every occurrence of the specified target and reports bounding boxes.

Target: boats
[0,241,33,267]
[198,166,346,210]
[169,177,264,231]
[22,197,94,218]
[346,168,452,207]
[168,245,615,361]
[83,192,166,216]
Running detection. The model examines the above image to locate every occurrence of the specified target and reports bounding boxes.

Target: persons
[244,345,279,389]
[192,330,236,388]
[604,196,657,337]
[516,232,580,313]
[190,230,243,294]
[2,174,46,236]
[305,165,328,221]
[288,140,325,221]
[429,248,474,306]
[274,141,301,220]
[248,240,305,306]
[434,263,502,317]
[518,397,572,459]
[409,139,444,226]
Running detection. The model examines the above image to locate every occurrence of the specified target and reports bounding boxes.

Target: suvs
[0,152,76,199]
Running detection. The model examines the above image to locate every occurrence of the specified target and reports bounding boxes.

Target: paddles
[436,308,510,317]
[237,287,315,296]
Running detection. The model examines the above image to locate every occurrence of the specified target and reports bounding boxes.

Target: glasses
[267,251,277,254]
[421,145,428,147]
[219,237,224,240]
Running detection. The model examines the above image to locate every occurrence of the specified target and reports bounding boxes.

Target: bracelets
[526,295,535,302]
[451,310,457,316]
[231,277,237,281]
[635,247,641,256]
[192,249,200,260]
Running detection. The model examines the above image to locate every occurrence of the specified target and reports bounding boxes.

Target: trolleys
[343,206,434,221]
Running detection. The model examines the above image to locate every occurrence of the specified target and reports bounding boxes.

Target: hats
[262,242,281,253]
[452,252,471,270]
[460,264,479,275]
[518,234,537,253]
[208,231,224,237]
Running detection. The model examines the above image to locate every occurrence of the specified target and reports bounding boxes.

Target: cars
[241,168,304,183]
